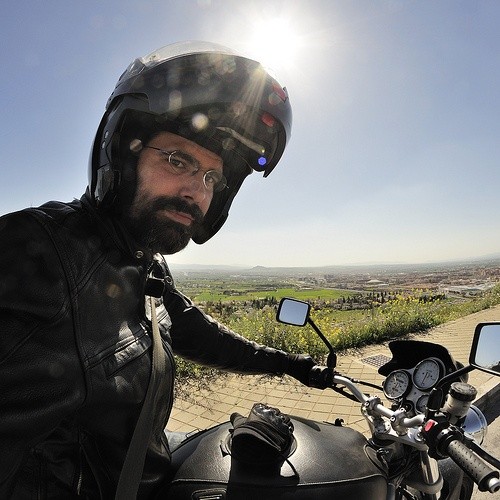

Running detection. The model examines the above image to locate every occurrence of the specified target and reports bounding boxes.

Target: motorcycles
[152,298,500,500]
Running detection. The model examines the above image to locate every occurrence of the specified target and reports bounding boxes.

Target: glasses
[138,143,232,192]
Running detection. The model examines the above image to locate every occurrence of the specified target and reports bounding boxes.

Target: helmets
[89,42,289,246]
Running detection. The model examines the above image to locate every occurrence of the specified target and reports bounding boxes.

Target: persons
[0,39,339,500]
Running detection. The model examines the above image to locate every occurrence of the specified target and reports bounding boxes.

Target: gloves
[284,352,317,386]
[229,402,295,466]
